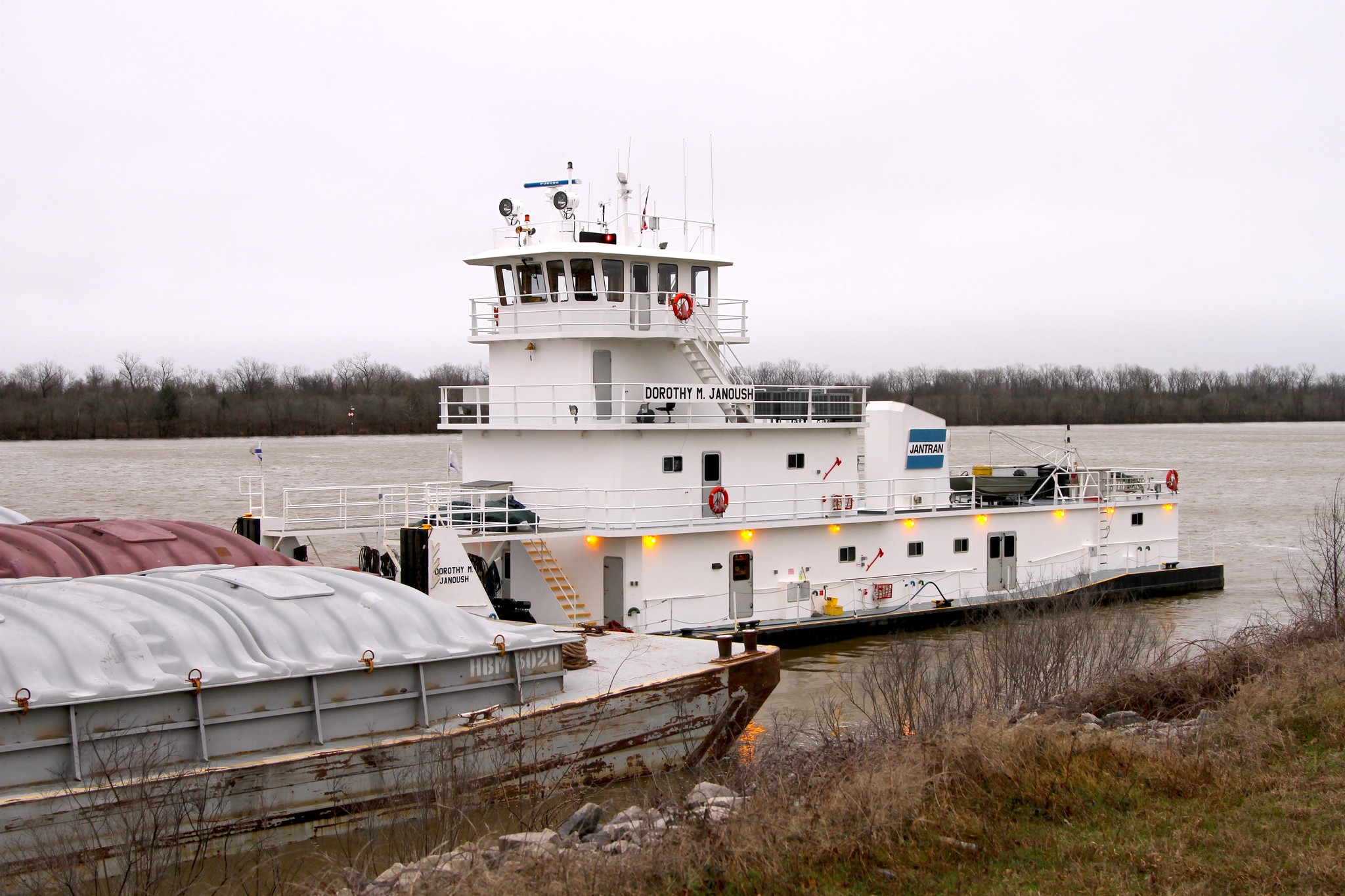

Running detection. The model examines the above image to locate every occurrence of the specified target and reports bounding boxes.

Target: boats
[2,3,1226,896]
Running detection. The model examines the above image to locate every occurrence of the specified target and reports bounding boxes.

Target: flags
[250,445,263,469]
[640,188,649,231]
[448,448,462,474]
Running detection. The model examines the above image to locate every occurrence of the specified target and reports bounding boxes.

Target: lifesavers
[708,486,729,515]
[672,292,693,321]
[1165,469,1179,491]
